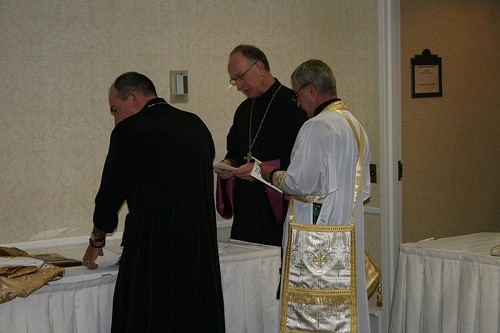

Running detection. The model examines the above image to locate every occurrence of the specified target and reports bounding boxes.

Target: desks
[0,231,281,333]
[389,231,500,333]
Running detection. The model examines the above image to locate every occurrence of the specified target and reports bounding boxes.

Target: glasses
[229,63,256,86]
[291,84,308,102]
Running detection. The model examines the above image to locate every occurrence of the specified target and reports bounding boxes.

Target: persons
[260,59,371,333]
[213,43,306,247]
[83,72,226,332]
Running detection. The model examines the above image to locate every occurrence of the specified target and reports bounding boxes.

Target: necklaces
[238,84,282,164]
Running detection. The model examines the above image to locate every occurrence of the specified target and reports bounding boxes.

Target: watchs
[90,231,105,242]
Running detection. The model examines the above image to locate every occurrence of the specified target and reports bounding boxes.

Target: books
[214,162,239,170]
[250,155,284,193]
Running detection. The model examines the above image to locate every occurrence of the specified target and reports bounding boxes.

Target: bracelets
[89,237,105,247]
[268,167,281,184]
